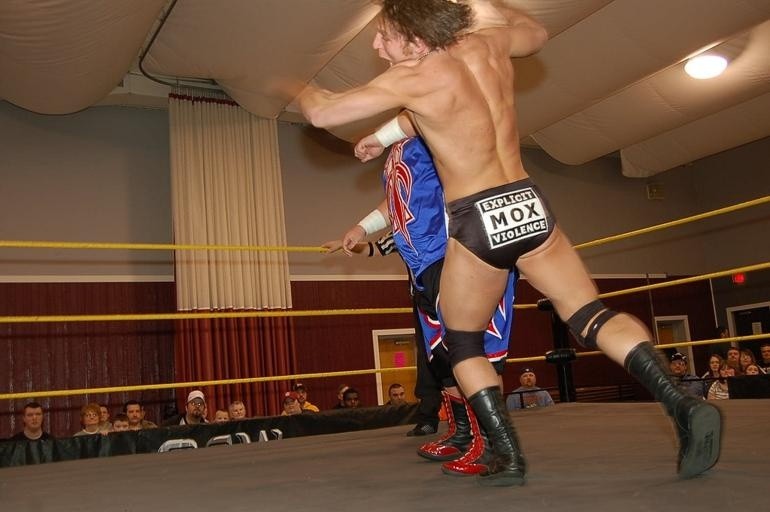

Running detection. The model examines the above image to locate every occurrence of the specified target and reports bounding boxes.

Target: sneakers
[407,422,437,436]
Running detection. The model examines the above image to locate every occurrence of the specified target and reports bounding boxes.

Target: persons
[280,382,319,416]
[8,401,58,443]
[382,383,407,406]
[286,0,722,485]
[333,382,362,410]
[506,365,555,413]
[72,399,157,437]
[320,229,443,436]
[344,106,520,477]
[157,390,247,427]
[667,341,770,400]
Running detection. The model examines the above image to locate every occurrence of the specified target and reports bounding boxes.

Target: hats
[187,390,205,405]
[282,391,298,403]
[670,353,688,363]
[293,382,306,392]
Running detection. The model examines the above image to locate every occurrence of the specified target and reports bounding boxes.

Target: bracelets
[373,116,410,148]
[357,209,387,236]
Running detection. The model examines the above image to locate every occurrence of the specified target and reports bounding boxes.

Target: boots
[624,341,721,480]
[417,385,527,487]
